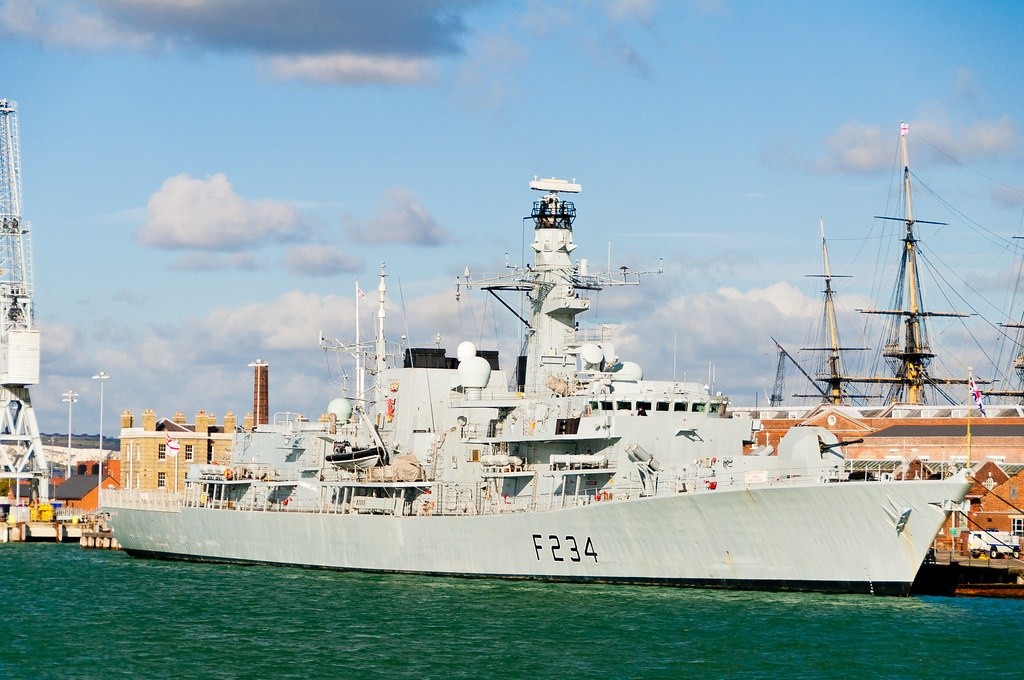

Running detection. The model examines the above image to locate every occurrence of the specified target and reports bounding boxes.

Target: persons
[637,407,647,416]
[586,446,592,454]
[344,444,352,454]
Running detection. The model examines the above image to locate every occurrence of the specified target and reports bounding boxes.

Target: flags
[970,375,985,417]
[166,436,180,457]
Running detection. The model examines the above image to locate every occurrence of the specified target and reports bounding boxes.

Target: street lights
[62,390,79,479]
[48,438,55,478]
[92,372,110,491]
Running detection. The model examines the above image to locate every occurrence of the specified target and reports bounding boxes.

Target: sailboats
[727,121,1024,551]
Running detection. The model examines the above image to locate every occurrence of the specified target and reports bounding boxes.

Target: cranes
[1,98,49,505]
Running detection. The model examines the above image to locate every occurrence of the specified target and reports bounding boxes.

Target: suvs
[968,529,1020,559]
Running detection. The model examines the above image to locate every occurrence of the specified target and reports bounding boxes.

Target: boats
[99,174,975,596]
[954,582,1024,598]
[547,454,609,470]
[326,439,382,471]
[479,454,524,468]
